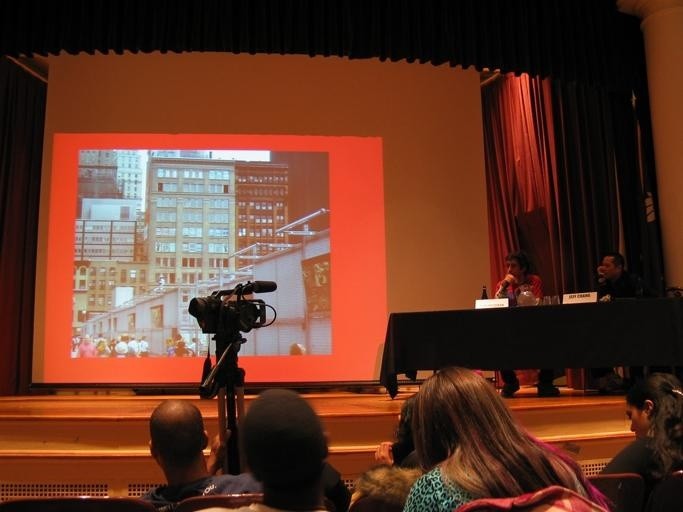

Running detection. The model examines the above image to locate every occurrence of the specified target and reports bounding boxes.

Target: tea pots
[513,284,536,306]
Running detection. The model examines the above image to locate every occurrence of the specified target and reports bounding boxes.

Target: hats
[81,334,91,340]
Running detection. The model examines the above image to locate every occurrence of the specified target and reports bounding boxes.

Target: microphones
[496,280,509,296]
[594,273,604,281]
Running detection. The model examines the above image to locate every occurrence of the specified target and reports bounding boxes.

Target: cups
[542,295,560,305]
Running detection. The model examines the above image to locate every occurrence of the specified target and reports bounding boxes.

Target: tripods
[217,367,246,475]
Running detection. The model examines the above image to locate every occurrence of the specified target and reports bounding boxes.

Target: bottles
[481,284,487,299]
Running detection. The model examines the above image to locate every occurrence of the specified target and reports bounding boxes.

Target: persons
[590,371,683,511]
[213,389,348,512]
[72,326,199,358]
[402,365,611,511]
[131,398,267,507]
[374,392,427,468]
[593,253,641,302]
[492,249,562,397]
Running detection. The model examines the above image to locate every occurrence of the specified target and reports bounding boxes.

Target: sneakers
[501,378,520,398]
[537,386,561,398]
